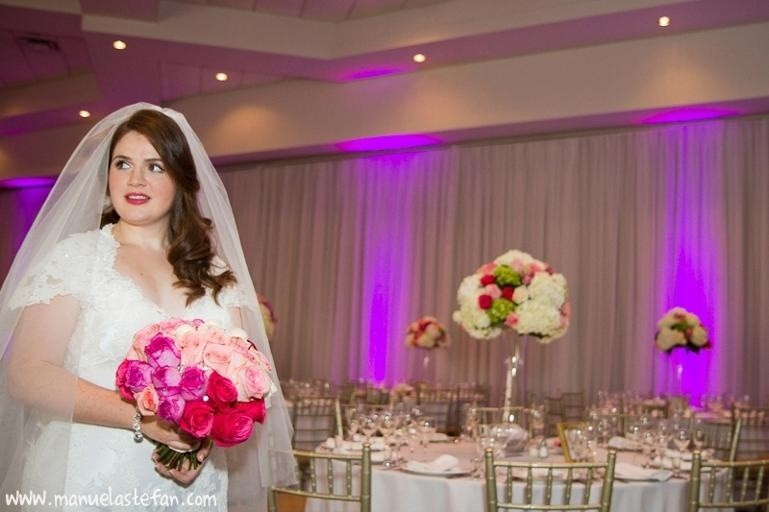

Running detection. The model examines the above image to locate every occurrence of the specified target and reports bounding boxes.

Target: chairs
[261,378,769,511]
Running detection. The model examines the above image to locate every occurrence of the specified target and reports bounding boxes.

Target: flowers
[114,318,277,470]
[404,315,450,352]
[653,305,712,357]
[450,249,570,344]
[255,291,278,337]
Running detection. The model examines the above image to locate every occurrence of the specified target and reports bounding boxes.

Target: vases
[495,332,528,456]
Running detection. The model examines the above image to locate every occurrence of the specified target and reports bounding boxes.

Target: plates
[320,432,404,463]
[401,462,471,477]
[610,434,714,484]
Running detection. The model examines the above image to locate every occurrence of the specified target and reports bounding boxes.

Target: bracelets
[132,408,144,445]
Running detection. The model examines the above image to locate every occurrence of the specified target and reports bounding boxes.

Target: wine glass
[466,407,525,479]
[630,413,705,479]
[342,399,438,470]
[562,425,605,482]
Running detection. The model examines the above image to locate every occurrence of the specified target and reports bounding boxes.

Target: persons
[0,98,302,512]
